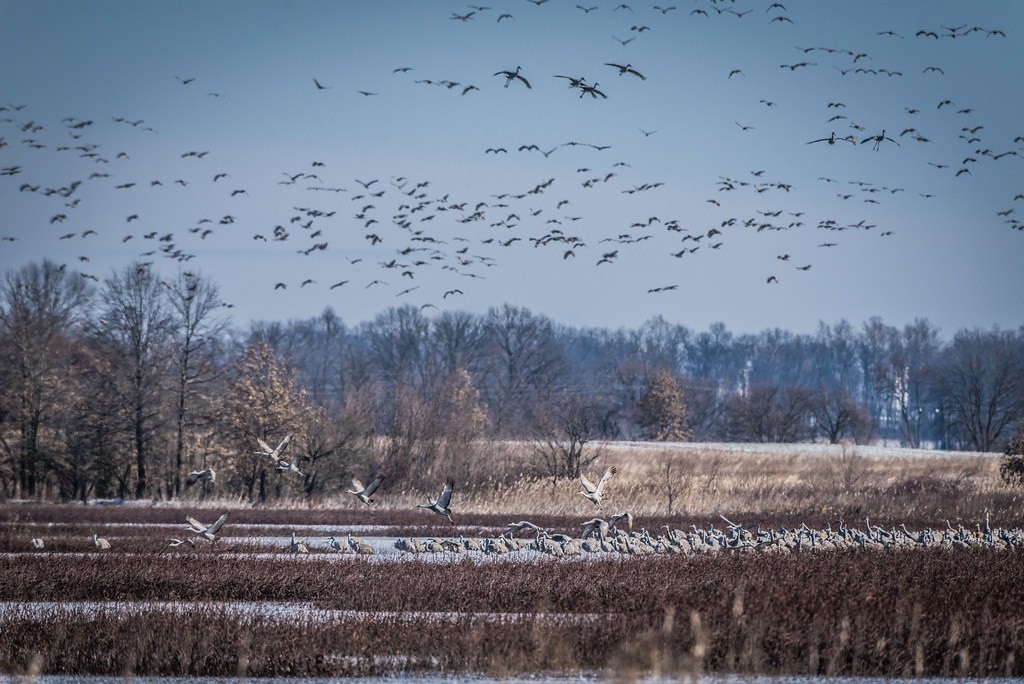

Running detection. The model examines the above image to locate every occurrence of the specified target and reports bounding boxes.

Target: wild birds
[0,0,1024,312]
[30,432,1023,552]
